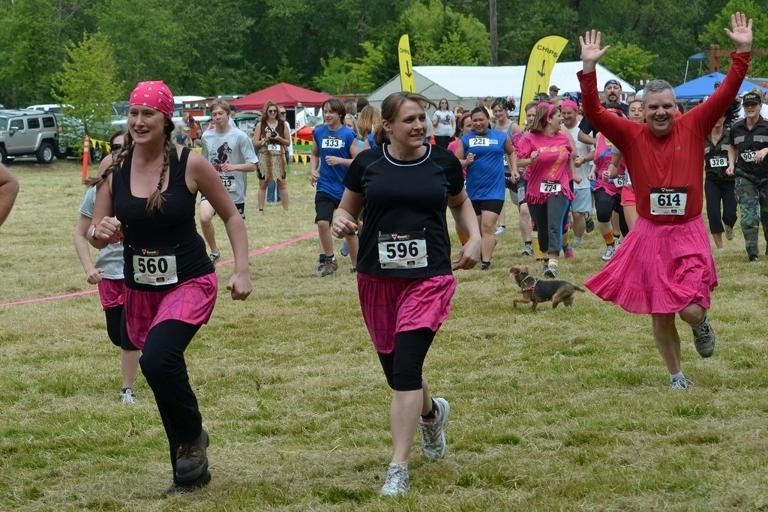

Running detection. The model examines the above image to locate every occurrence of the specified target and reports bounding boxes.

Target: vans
[96,104,118,115]
[170,94,204,116]
[26,104,73,113]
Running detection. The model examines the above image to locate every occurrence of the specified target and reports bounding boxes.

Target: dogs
[509,266,586,314]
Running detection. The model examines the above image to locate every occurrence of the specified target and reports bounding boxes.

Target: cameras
[271,132,276,138]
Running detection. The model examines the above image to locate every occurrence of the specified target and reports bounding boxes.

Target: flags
[518,35,570,129]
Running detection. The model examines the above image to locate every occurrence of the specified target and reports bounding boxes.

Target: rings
[586,42,590,45]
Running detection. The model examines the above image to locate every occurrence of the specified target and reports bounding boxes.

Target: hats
[549,85,560,91]
[743,92,761,104]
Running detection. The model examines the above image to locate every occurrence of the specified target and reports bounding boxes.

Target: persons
[429,81,645,275]
[174,97,383,277]
[703,87,768,261]
[87,80,253,498]
[331,91,483,497]
[71,128,140,407]
[0,162,19,225]
[575,11,754,391]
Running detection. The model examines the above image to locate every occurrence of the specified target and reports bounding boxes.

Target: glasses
[744,103,758,108]
[268,110,277,113]
[553,90,558,92]
[110,144,121,152]
[281,112,286,115]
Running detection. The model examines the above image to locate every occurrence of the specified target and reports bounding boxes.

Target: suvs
[0,110,58,163]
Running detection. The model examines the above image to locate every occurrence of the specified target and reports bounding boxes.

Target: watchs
[91,223,99,241]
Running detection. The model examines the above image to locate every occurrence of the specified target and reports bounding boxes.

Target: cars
[91,115,129,132]
[53,121,106,161]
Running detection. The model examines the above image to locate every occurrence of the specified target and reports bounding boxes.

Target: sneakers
[318,257,338,277]
[175,430,210,483]
[493,224,734,279]
[340,245,349,256]
[668,376,694,390]
[691,316,715,357]
[380,462,410,496]
[418,397,449,460]
[209,251,220,263]
[119,387,136,405]
[168,469,211,494]
